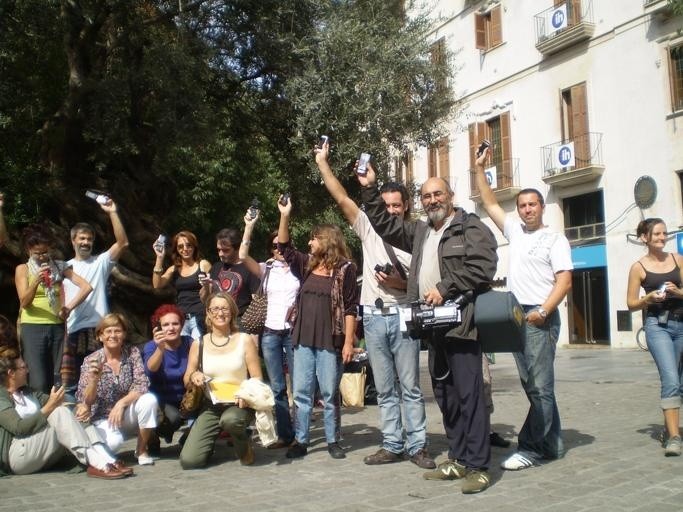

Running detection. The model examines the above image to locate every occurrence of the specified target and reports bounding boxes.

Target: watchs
[154,265,164,273]
[241,240,250,246]
[537,307,549,319]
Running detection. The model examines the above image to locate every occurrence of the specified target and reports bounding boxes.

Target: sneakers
[461,469,490,493]
[423,460,469,479]
[664,436,682,455]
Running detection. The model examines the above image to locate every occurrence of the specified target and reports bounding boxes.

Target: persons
[472,139,574,472]
[354,159,500,495]
[277,193,359,460]
[198,228,261,318]
[626,216,683,457]
[238,205,300,450]
[14,224,93,393]
[314,139,438,471]
[62,195,130,400]
[178,291,265,470]
[141,303,197,461]
[151,230,212,321]
[74,314,158,465]
[0,345,134,480]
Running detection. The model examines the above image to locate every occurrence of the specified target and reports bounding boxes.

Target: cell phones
[477,139,490,158]
[41,263,49,269]
[204,375,213,383]
[199,272,207,278]
[155,235,166,251]
[86,191,108,205]
[251,206,256,218]
[356,153,371,174]
[158,320,161,331]
[316,135,328,152]
[279,192,292,206]
[54,373,62,393]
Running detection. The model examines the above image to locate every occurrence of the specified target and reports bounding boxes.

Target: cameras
[97,352,108,372]
[410,294,469,331]
[660,284,666,293]
[374,262,395,276]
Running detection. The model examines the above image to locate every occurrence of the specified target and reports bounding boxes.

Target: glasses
[30,252,50,261]
[177,245,193,250]
[208,306,230,313]
[11,365,30,370]
[270,243,278,249]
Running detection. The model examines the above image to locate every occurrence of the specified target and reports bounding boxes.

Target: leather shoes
[410,448,436,468]
[286,444,308,457]
[114,460,134,476]
[87,462,125,479]
[499,450,541,469]
[134,448,154,465]
[327,442,345,459]
[363,448,406,464]
[489,433,511,446]
[242,445,256,465]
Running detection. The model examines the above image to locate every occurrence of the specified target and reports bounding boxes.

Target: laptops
[207,382,240,406]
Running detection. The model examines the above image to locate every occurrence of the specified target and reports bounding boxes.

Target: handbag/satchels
[241,262,269,334]
[341,363,368,409]
[180,336,207,414]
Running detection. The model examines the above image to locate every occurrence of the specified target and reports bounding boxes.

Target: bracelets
[62,307,68,315]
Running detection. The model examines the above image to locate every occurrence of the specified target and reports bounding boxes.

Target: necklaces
[10,391,26,406]
